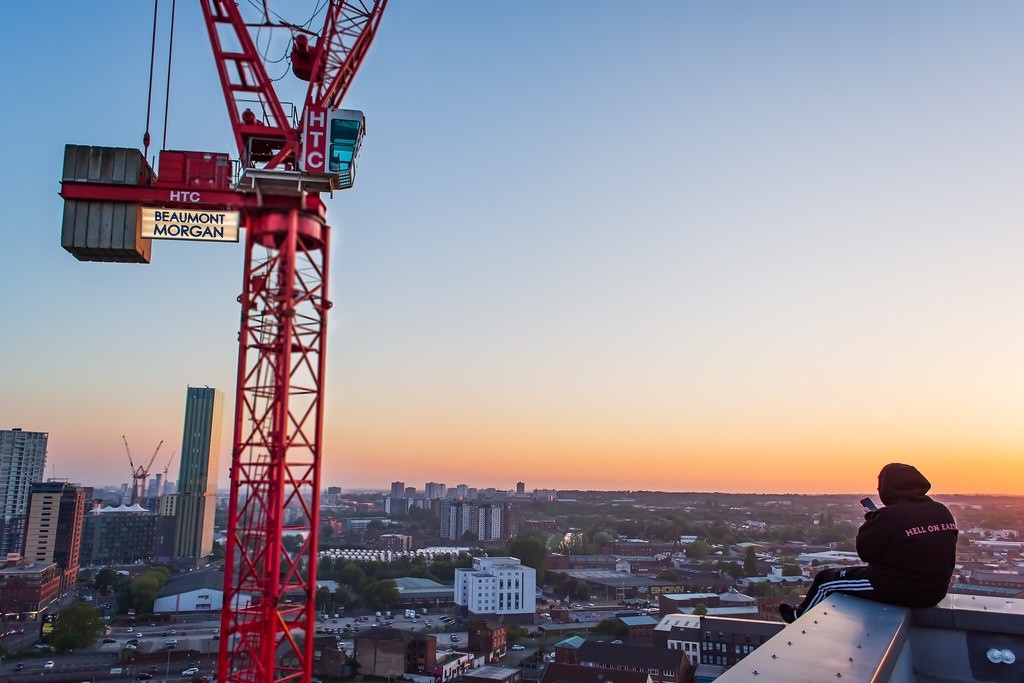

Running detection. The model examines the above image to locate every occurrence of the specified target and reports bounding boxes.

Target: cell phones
[860,497,877,511]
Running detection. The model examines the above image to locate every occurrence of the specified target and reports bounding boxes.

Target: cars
[16,662,24,671]
[181,668,199,677]
[281,584,660,667]
[44,660,54,669]
[0,555,220,653]
[135,673,152,681]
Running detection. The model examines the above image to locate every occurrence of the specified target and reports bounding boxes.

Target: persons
[780,463,958,624]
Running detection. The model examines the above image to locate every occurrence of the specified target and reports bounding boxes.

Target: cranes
[122,435,163,507]
[164,450,175,493]
[57,0,389,683]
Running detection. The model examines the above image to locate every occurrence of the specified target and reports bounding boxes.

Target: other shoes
[779,603,796,624]
